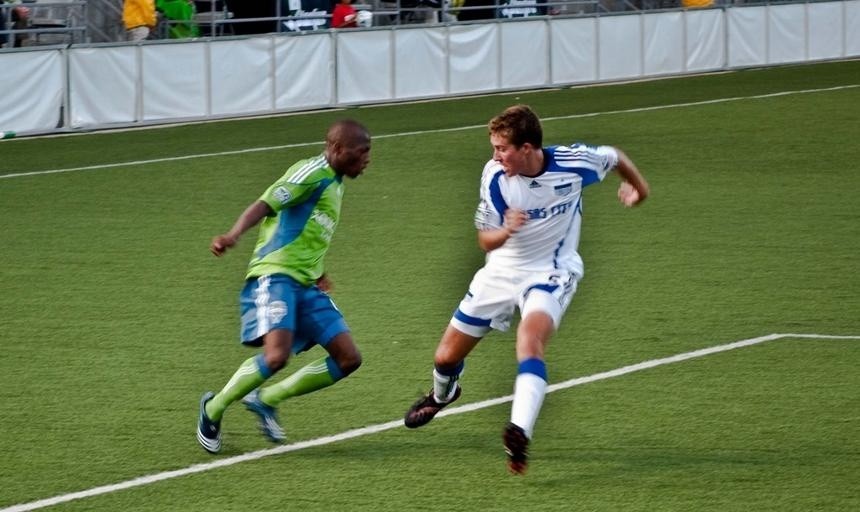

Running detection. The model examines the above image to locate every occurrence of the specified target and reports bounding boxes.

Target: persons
[121,0,560,41]
[0,0,30,48]
[404,105,649,473]
[196,120,372,454]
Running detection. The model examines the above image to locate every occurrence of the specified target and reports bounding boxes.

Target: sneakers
[503,422,529,475]
[405,380,461,430]
[197,391,222,453]
[242,389,288,440]
